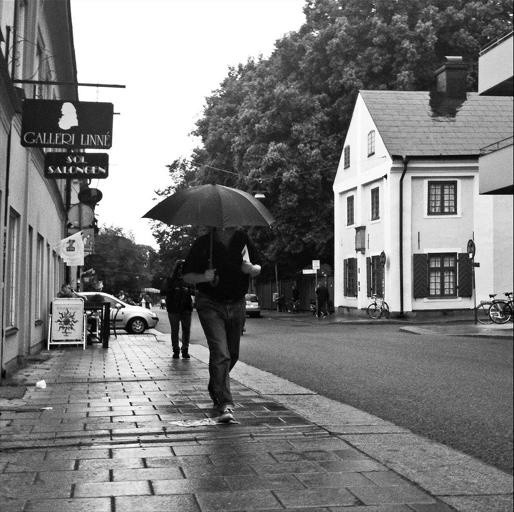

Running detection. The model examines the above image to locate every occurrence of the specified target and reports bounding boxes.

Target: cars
[76,291,160,333]
[245,293,261,315]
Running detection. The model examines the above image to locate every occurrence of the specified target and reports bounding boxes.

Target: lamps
[67,223,74,228]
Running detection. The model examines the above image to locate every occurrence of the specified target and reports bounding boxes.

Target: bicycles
[476,292,514,325]
[364,293,392,320]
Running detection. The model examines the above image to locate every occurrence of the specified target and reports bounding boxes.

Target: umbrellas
[140,181,275,286]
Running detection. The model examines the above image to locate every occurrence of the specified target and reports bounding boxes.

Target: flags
[48,229,84,266]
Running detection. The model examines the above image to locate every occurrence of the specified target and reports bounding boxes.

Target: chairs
[105,304,127,339]
[81,310,100,344]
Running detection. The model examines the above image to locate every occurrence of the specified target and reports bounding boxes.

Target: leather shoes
[173,353,179,358]
[182,353,190,358]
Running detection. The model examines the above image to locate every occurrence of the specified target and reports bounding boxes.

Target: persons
[314,282,328,318]
[180,227,261,421]
[55,281,303,314]
[158,260,194,359]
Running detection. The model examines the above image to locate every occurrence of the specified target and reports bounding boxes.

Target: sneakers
[219,403,236,423]
